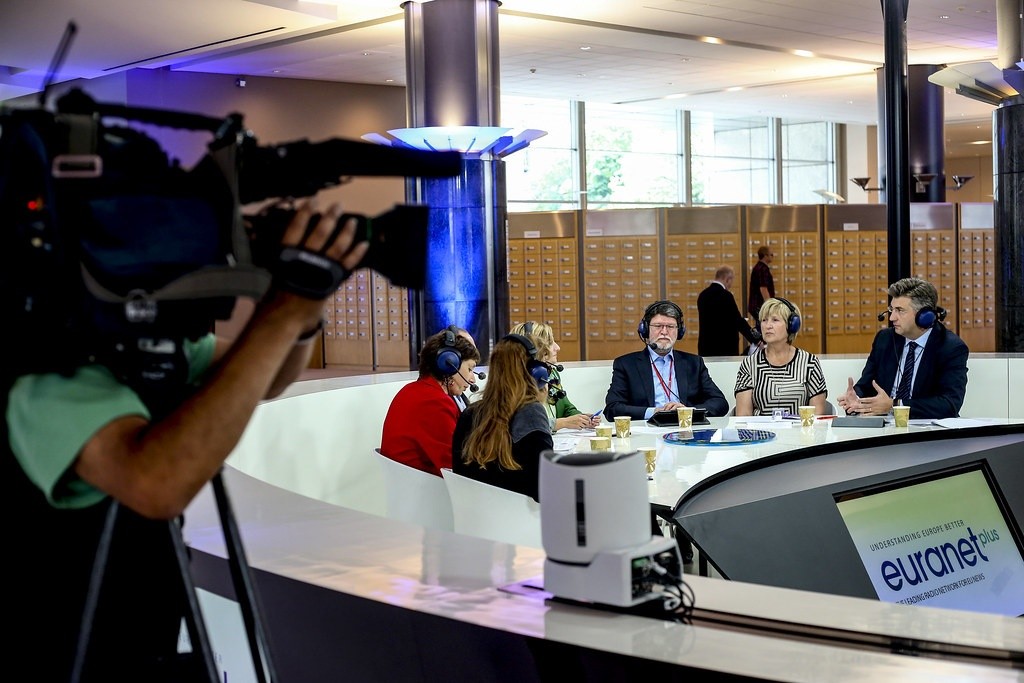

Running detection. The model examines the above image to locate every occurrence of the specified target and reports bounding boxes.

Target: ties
[895,341,918,400]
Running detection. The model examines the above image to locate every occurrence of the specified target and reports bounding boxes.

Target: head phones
[501,334,549,389]
[915,306,947,329]
[756,297,801,334]
[639,301,686,340]
[434,330,462,376]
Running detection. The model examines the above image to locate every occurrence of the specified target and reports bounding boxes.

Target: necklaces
[767,347,791,406]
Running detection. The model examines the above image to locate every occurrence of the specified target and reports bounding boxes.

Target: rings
[870,408,871,413]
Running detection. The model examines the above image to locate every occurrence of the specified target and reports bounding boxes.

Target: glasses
[649,324,677,332]
[887,307,917,316]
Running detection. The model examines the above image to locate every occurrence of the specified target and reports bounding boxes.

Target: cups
[614,415,631,439]
[798,405,816,427]
[589,437,609,452]
[892,406,911,427]
[594,425,613,448]
[679,429,694,441]
[637,447,657,481]
[677,407,693,429]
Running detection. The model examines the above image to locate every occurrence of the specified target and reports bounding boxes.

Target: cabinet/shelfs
[822,201,887,353]
[909,200,958,334]
[662,203,745,354]
[743,201,824,355]
[507,207,581,361]
[321,269,371,372]
[583,204,664,359]
[958,200,995,353]
[369,269,410,373]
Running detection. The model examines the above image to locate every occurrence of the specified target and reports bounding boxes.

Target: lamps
[947,174,975,191]
[912,172,938,194]
[849,177,884,192]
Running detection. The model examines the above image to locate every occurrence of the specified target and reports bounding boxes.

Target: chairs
[727,399,835,418]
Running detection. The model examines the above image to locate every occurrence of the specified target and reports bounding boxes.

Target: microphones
[750,327,761,339]
[454,366,479,393]
[473,371,486,380]
[878,310,889,321]
[295,137,464,177]
[638,333,657,350]
[547,361,564,372]
[543,380,566,399]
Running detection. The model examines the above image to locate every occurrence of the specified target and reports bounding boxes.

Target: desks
[554,417,1024,576]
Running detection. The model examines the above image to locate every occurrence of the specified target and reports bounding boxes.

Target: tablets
[647,408,707,423]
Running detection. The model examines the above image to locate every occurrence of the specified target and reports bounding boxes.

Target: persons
[697,265,759,356]
[603,300,730,417]
[0,198,369,683]
[509,321,600,430]
[838,278,968,419]
[440,325,477,410]
[748,247,775,324]
[452,336,553,503]
[733,297,828,414]
[380,332,480,476]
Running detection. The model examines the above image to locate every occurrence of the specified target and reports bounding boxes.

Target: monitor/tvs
[837,460,1024,619]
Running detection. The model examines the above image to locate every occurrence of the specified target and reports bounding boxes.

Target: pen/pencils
[817,416,838,419]
[590,409,602,420]
[783,417,800,420]
